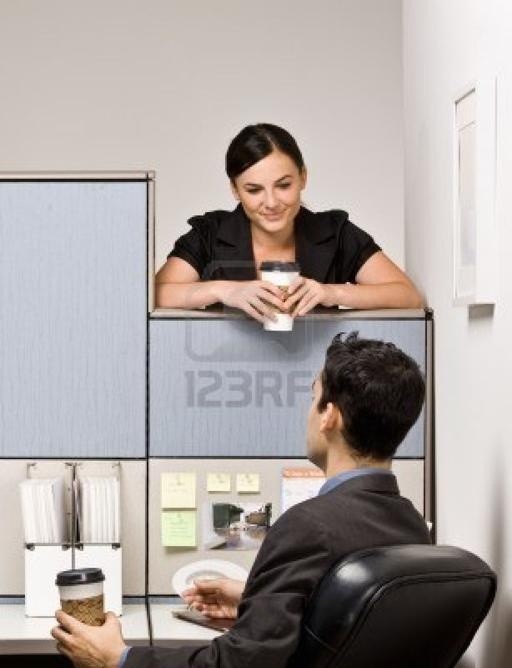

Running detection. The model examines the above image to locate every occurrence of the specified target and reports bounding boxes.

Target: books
[19,461,121,544]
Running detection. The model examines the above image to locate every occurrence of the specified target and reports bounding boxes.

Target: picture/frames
[451,78,497,307]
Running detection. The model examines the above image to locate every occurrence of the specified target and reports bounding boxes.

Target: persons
[51,332,433,668]
[154,123,421,325]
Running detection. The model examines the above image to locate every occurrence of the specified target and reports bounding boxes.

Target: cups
[260,261,300,333]
[55,568,105,633]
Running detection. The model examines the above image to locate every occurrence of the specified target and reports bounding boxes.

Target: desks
[0,602,151,655]
[147,604,229,651]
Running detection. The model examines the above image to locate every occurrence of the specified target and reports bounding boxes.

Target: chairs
[290,544,496,668]
